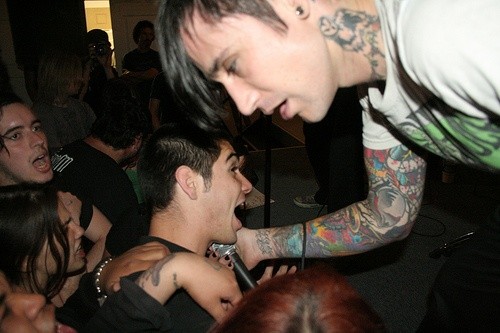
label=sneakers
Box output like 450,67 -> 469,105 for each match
293,194 -> 328,209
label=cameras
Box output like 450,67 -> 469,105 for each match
93,42 -> 107,57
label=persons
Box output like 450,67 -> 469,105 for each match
154,0 -> 500,333
0,19 -> 389,333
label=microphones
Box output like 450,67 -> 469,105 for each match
213,241 -> 259,293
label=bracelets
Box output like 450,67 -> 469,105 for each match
95,258 -> 113,298
